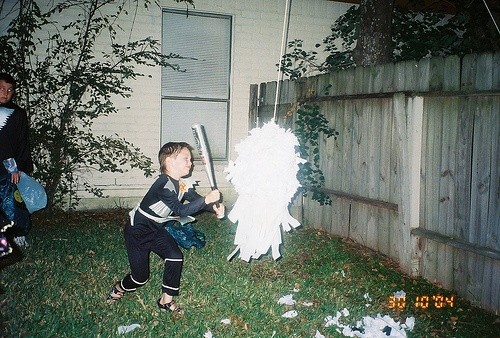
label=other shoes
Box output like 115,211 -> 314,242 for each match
13,235 -> 29,248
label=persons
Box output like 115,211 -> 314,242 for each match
105,142 -> 225,316
0,72 -> 33,249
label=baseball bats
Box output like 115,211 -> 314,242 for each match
193,123 -> 225,218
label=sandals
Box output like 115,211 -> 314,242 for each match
157,298 -> 182,316
107,281 -> 125,304
